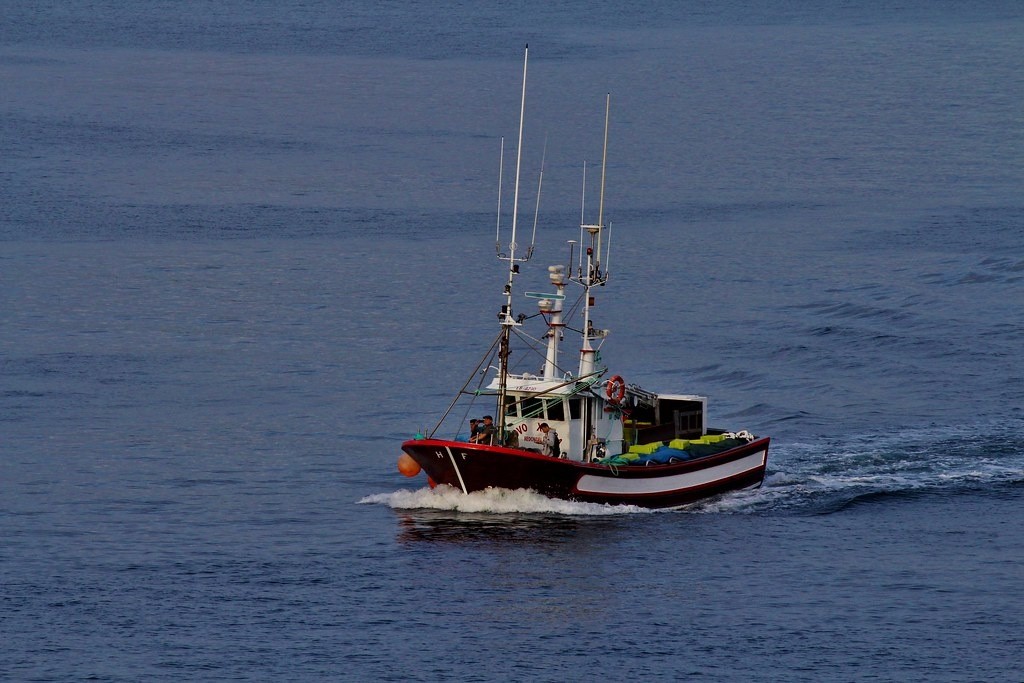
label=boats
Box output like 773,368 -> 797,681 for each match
395,41 -> 771,508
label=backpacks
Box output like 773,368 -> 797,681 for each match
550,433 -> 560,457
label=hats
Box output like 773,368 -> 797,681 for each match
539,423 -> 548,428
483,415 -> 492,421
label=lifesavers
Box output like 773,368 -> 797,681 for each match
606,375 -> 625,402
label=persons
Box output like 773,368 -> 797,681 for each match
468,415 -> 498,446
540,423 -> 560,458
